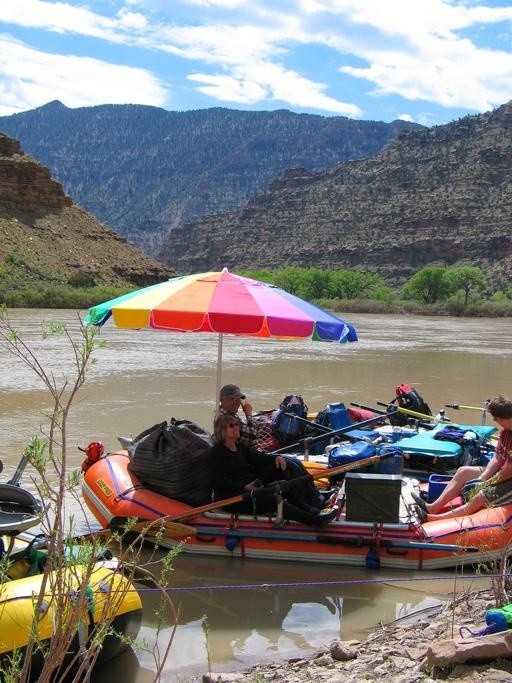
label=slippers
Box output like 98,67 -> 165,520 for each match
413,506 -> 428,523
411,492 -> 430,513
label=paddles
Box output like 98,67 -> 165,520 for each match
285,412 -> 447,476
66,452 -> 395,543
15,532 -> 157,588
119,521 -> 479,553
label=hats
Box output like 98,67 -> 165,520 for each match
219,383 -> 246,401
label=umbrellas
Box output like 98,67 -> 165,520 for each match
83,266 -> 358,422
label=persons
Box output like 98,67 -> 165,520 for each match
214,384 -> 259,449
410,396 -> 512,524
210,415 -> 338,529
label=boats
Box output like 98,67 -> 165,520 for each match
0,511 -> 145,683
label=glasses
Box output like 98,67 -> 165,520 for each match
228,422 -> 237,427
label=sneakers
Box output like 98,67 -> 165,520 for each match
320,488 -> 335,500
317,509 -> 339,524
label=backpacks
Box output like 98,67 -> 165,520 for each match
271,394 -> 308,435
